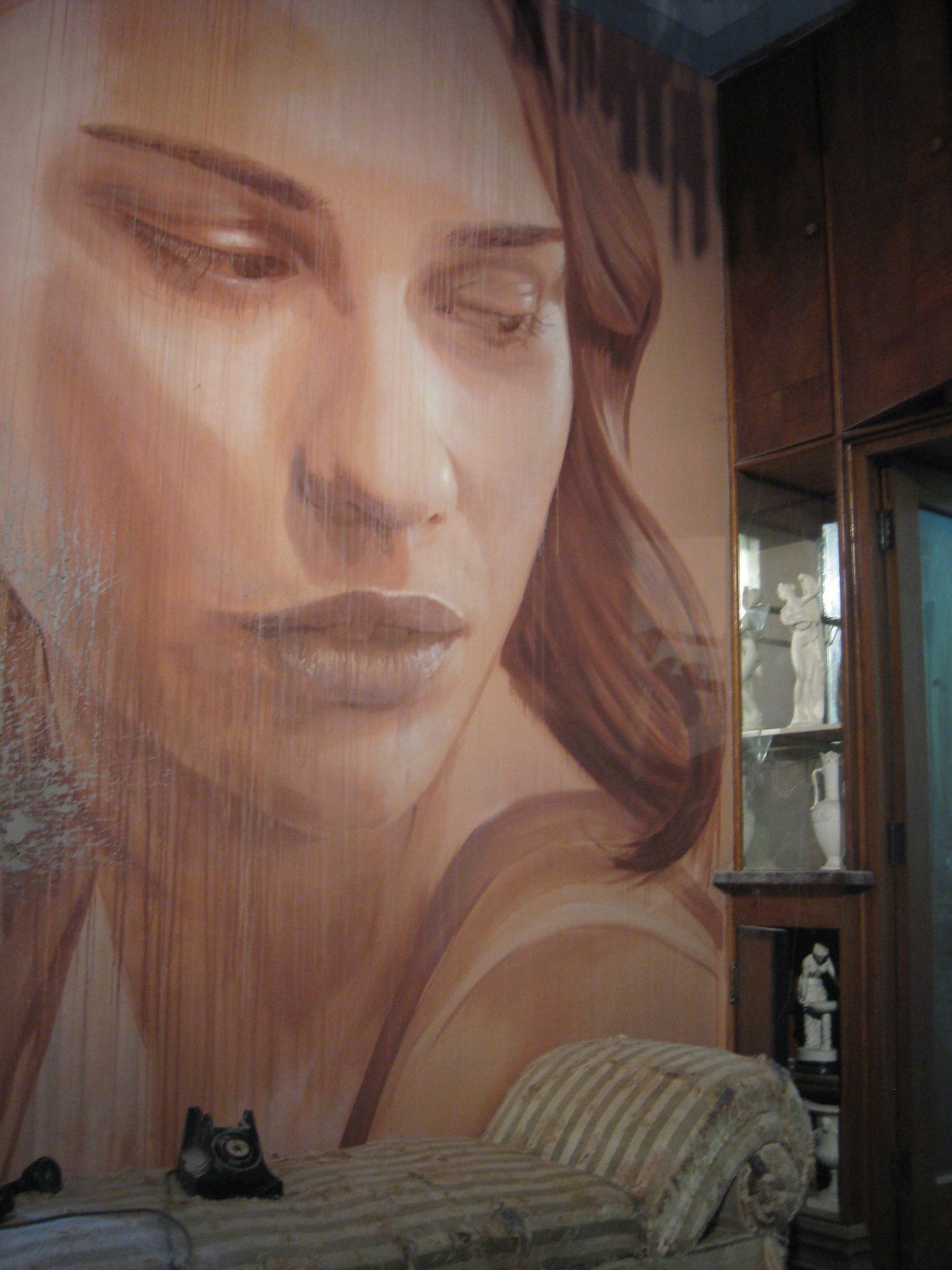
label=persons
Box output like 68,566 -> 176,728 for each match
739,586 -> 770,731
798,945 -> 837,1050
776,582 -> 824,728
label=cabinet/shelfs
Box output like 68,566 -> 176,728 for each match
774,927 -> 849,1236
736,441 -> 842,867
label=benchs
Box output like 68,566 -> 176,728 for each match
1,1036 -> 815,1270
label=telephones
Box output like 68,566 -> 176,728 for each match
0,1156 -> 64,1217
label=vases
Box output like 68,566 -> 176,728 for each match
810,748 -> 842,872
